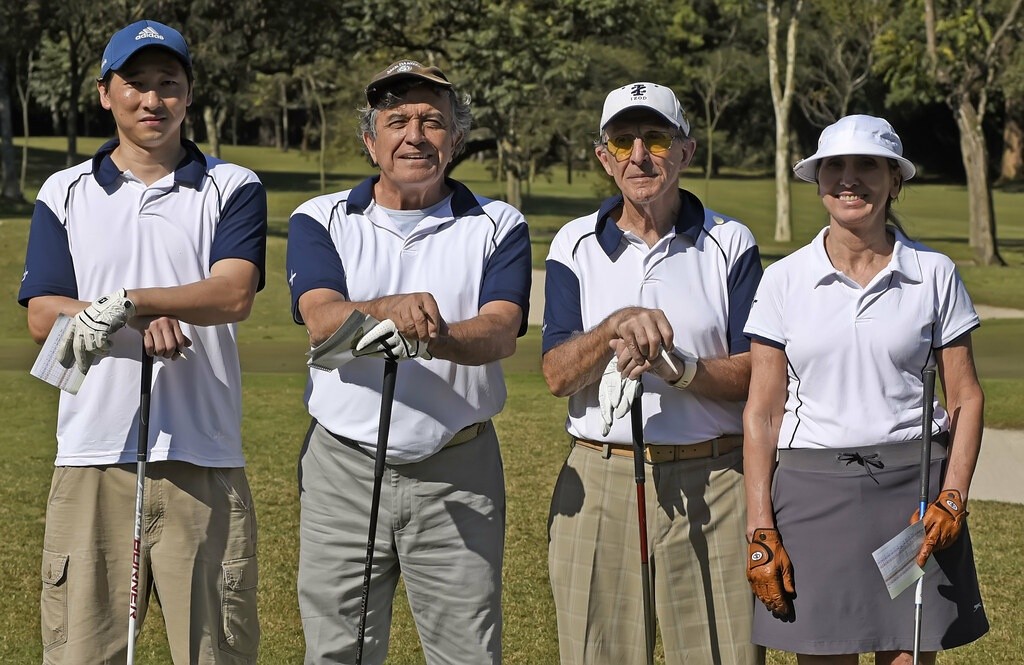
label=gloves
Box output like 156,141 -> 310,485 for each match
900,488 -> 971,566
353,317 -> 432,362
55,286 -> 138,375
741,526 -> 798,620
600,356 -> 642,438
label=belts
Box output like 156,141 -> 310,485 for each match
441,416 -> 488,450
575,438 -> 746,463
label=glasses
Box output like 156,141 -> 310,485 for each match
602,131 -> 675,156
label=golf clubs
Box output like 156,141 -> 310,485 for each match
912,367 -> 937,665
632,380 -> 658,664
122,334 -> 155,665
356,358 -> 399,664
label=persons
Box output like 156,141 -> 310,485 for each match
742,114 -> 990,665
541,81 -> 764,665
17,19 -> 267,665
285,58 -> 532,665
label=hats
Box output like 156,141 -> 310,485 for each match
365,58 -> 457,121
793,113 -> 917,184
600,81 -> 691,136
98,18 -> 196,87
